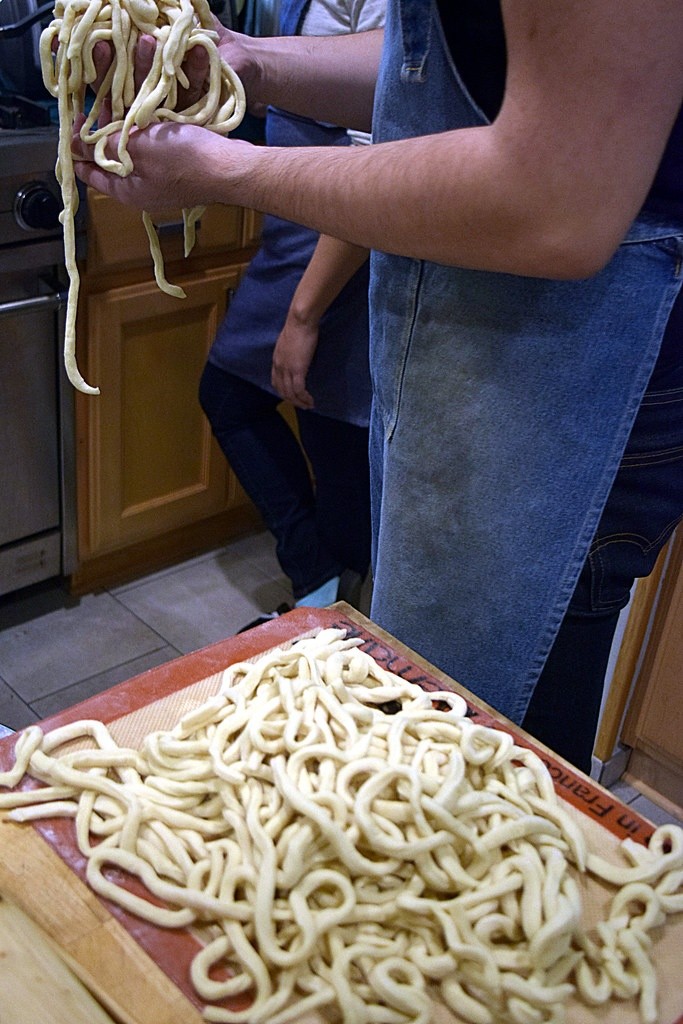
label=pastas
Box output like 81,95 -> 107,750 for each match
40,0 -> 245,395
0,626 -> 682,1024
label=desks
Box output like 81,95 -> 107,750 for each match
1,600 -> 683,1024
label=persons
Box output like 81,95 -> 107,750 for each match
197,0 -> 402,634
66,0 -> 683,787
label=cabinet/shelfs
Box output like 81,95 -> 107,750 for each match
76,186 -> 316,595
